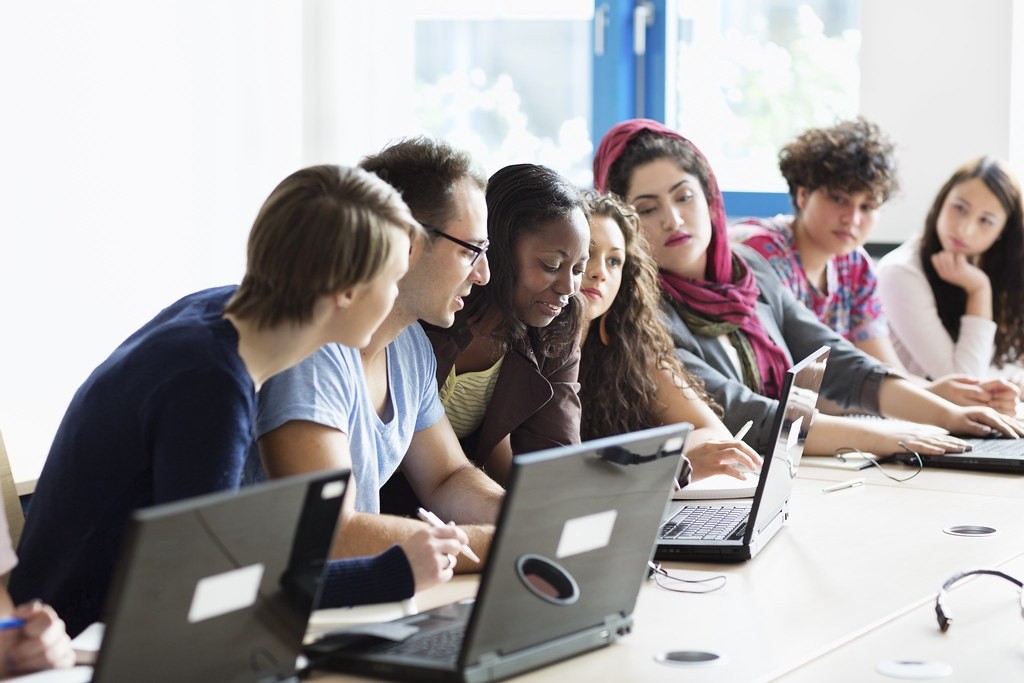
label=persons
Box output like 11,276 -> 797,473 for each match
720,117 -> 1022,421
0,117 -> 1024,674
874,154 -> 1023,382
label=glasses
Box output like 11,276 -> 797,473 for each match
418,223 -> 491,267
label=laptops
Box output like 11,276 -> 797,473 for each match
652,344 -> 830,564
90,465 -> 350,683
302,422 -> 692,683
896,432 -> 1024,471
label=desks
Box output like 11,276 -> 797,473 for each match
0,453 -> 1024,683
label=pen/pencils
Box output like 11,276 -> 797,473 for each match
414,505 -> 482,568
822,477 -> 865,493
0,617 -> 26,631
732,418 -> 755,443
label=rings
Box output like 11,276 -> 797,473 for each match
444,554 -> 452,570
